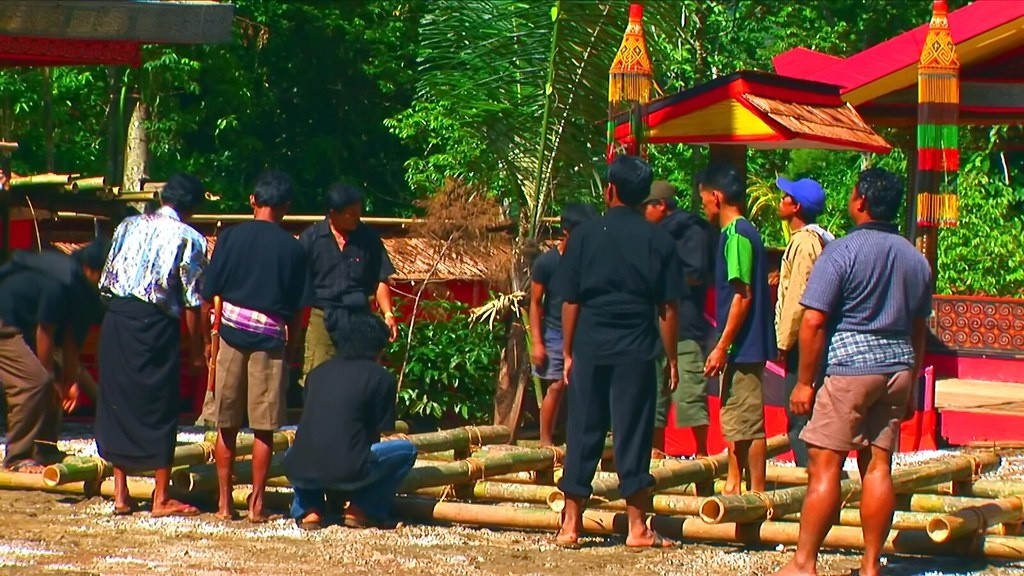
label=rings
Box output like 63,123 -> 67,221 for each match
719,371 -> 722,375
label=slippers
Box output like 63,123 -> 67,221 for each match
112,499 -> 138,514
211,510 -> 233,522
548,532 -> 581,550
297,511 -> 322,530
12,461 -> 41,474
342,511 -> 367,529
246,512 -> 268,523
625,529 -> 683,553
151,503 -> 201,518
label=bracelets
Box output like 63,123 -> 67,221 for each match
384,312 -> 394,317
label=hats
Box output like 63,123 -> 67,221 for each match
776,177 -> 826,215
643,180 -> 675,204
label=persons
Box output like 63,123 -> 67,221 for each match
96,173 -> 208,518
768,167 -> 932,576
556,156 -> 680,547
298,182 -> 398,389
0,237 -> 112,474
282,313 -> 419,530
529,167 -> 835,498
200,173 -> 307,523
